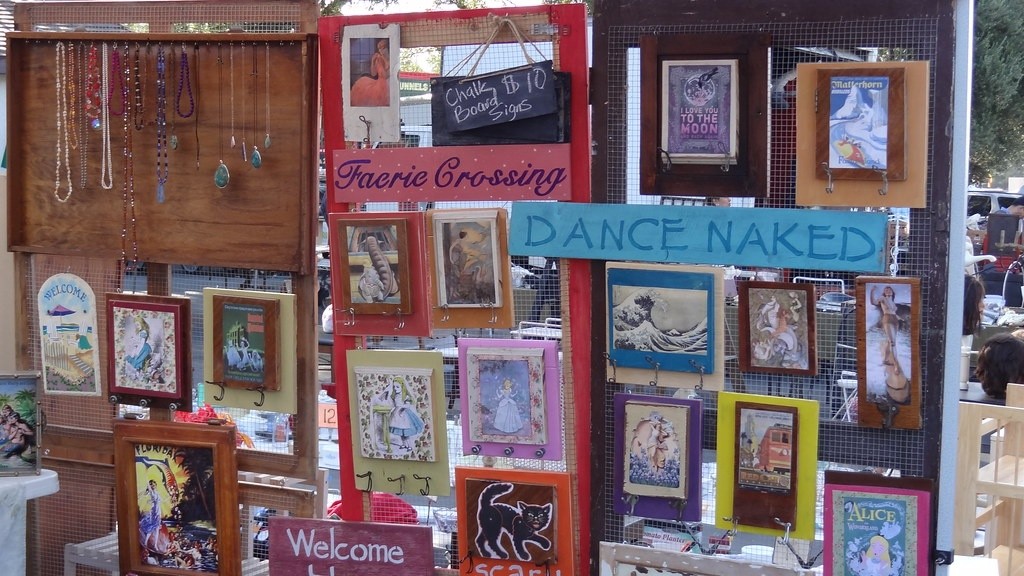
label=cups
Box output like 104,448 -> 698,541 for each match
740,545 -> 774,562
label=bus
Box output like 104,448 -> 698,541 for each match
757,424 -> 792,473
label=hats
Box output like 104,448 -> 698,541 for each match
967,214 -> 981,230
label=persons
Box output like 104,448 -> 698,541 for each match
995,198 -> 1024,220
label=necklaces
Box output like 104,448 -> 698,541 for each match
156,46 -> 169,204
54,38 -> 275,205
175,43 -> 196,118
121,46 -> 139,272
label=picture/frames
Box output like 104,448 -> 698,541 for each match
212,294 -> 281,392
336,218 -> 412,315
815,67 -> 906,182
112,417 -> 241,576
107,298 -> 183,399
429,210 -> 504,309
467,348 -> 547,444
738,280 -> 819,376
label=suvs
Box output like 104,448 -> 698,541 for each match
966,189 -> 1024,229
